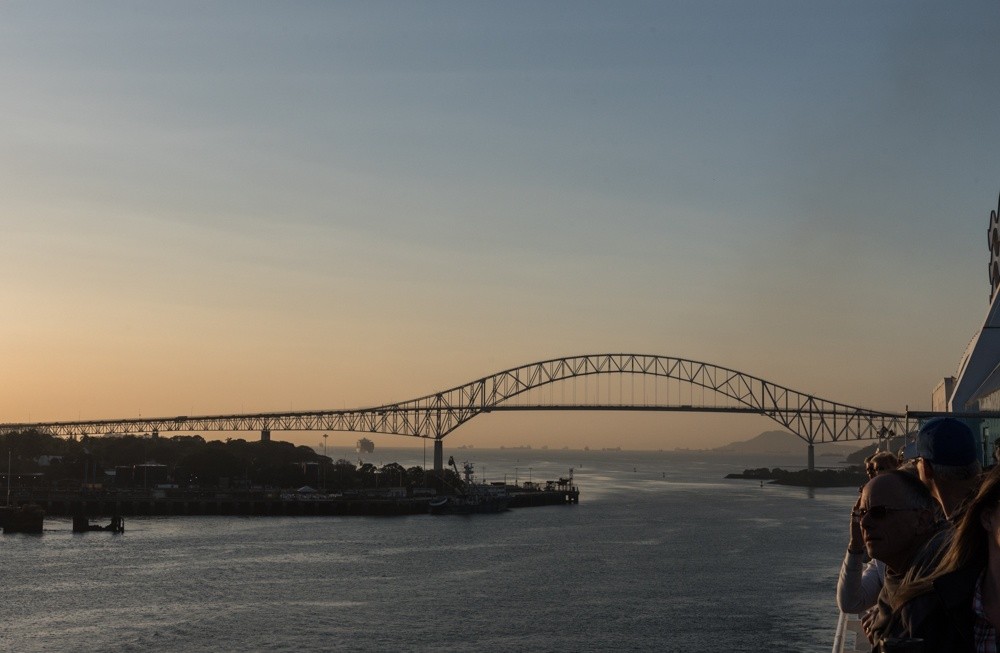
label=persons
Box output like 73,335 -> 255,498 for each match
835,418 -> 1000,614
856,468 -> 955,645
872,463 -> 1000,653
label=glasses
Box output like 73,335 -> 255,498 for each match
865,467 -> 875,472
874,466 -> 890,471
852,504 -> 922,523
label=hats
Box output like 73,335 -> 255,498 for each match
906,418 -> 978,465
